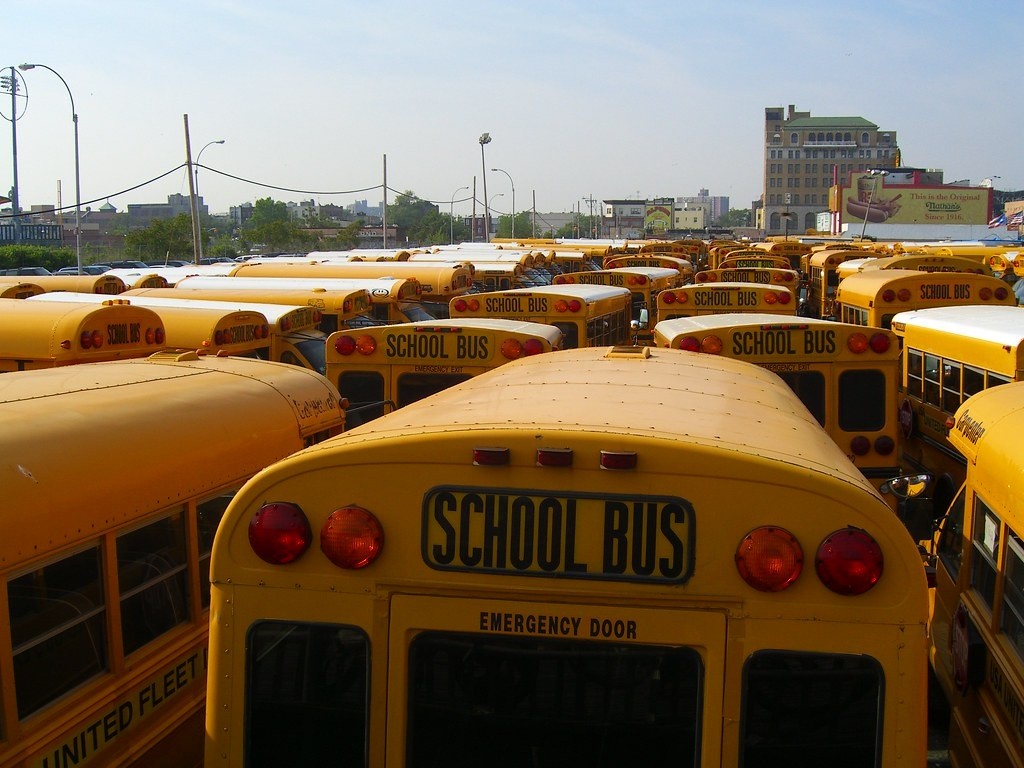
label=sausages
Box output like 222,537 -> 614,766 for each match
847,196 -> 891,222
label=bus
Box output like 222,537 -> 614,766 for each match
1,236 -> 1024,768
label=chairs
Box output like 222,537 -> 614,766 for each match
79,547 -> 187,656
7,584 -> 104,720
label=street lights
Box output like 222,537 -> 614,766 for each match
18,62 -> 84,274
195,139 -> 226,260
479,133 -> 493,242
489,168 -> 515,238
487,193 -> 503,241
449,185 -> 469,244
